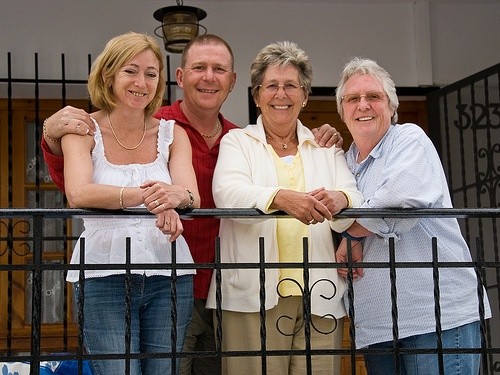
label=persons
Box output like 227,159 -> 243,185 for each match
335,57 -> 492,375
61,31 -> 202,375
205,42 -> 362,375
41,35 -> 343,375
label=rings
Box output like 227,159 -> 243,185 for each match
307,219 -> 315,225
166,218 -> 170,225
155,200 -> 159,206
334,133 -> 338,139
77,120 -> 81,128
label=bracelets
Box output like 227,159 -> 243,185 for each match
120,188 -> 124,209
342,231 -> 365,241
43,118 -> 61,143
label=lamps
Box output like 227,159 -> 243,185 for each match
153,0 -> 208,54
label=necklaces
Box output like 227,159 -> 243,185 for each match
107,110 -> 146,149
265,134 -> 295,150
200,118 -> 221,138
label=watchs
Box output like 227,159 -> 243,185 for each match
177,190 -> 194,210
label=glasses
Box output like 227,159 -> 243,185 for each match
340,92 -> 388,106
259,80 -> 304,91
